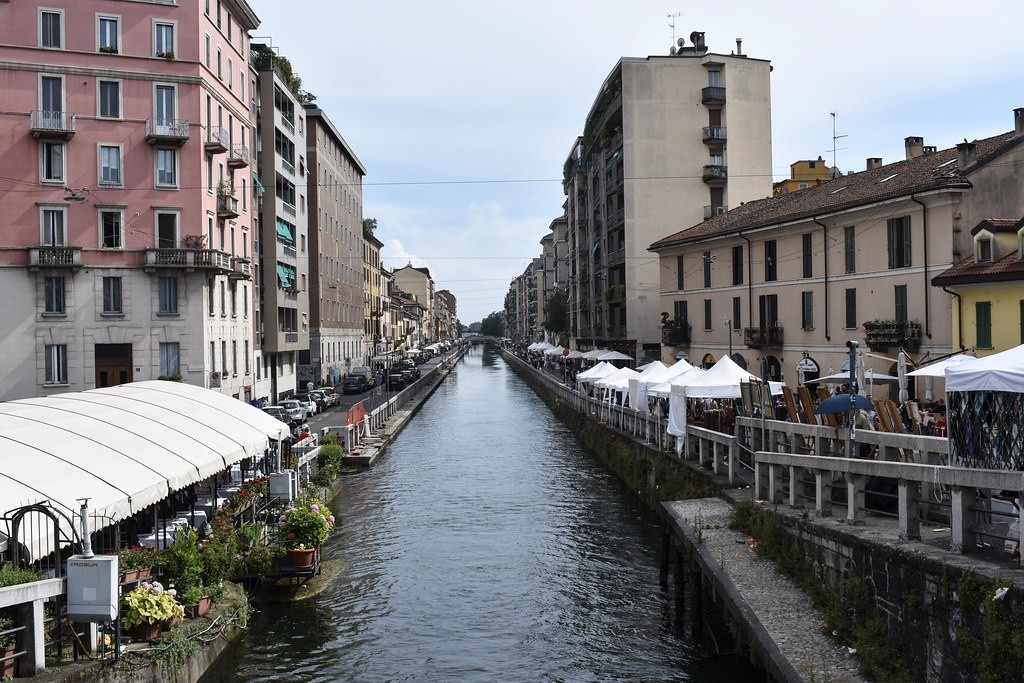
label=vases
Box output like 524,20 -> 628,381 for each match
132,622 -> 162,640
137,568 -> 151,577
166,617 -> 182,630
286,545 -> 315,567
122,571 -> 138,582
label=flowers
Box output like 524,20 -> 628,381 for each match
121,579 -> 185,625
106,543 -> 162,569
209,475 -> 335,549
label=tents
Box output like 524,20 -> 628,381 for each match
527,342 -> 634,369
576,354 -> 785,421
0,380 -> 291,566
944,343 -> 1024,466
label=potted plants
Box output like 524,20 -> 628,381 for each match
168,525 -> 213,616
319,445 -> 343,479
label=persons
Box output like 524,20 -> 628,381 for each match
835,386 -> 842,395
250,397 -> 268,409
307,381 -> 313,392
854,408 -> 879,458
933,399 -> 947,437
841,384 -> 848,394
297,427 -> 314,442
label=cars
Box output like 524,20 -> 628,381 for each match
343,364 -> 421,395
261,406 -> 292,424
311,393 -> 327,414
310,390 -> 332,408
278,400 -> 308,425
291,393 -> 317,417
319,386 -> 341,406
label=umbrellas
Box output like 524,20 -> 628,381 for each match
814,394 -> 874,424
904,354 -> 978,378
802,349 -> 912,404
